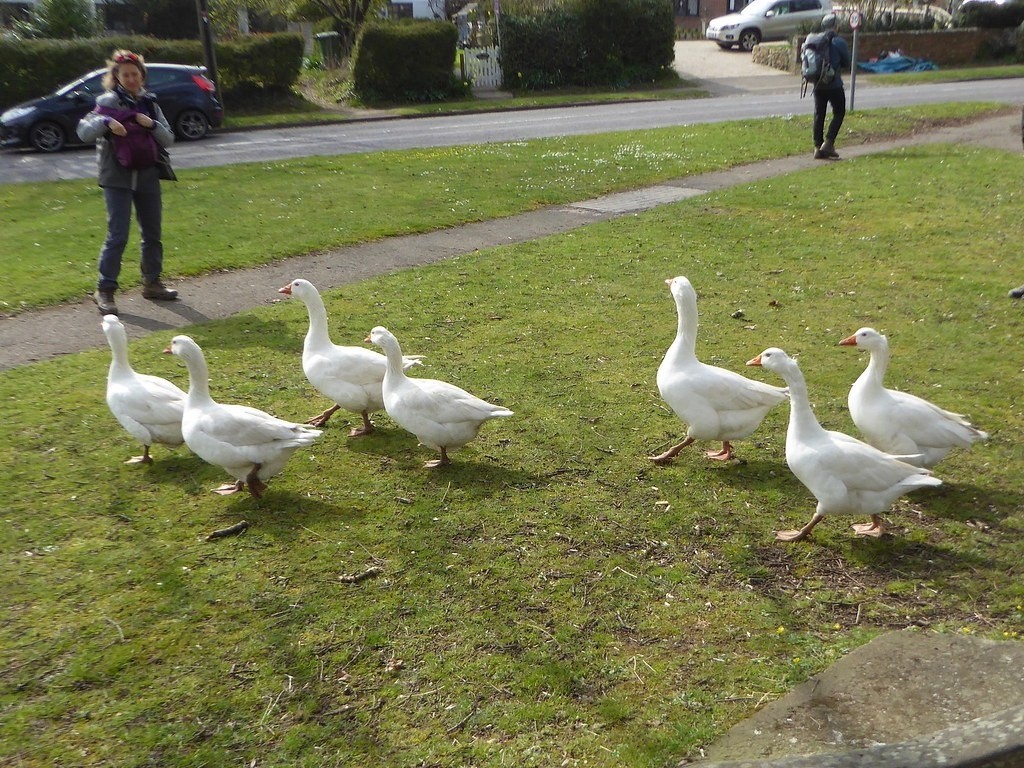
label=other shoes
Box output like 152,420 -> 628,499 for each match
819,139 -> 839,158
813,146 -> 826,158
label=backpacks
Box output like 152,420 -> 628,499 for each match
799,31 -> 835,85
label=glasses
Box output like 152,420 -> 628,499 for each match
113,52 -> 138,63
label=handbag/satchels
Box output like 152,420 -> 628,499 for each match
92,105 -> 157,169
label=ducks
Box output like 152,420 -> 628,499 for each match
642,276 -> 792,463
162,334 -> 324,499
747,346 -> 943,541
280,276 -> 428,437
839,326 -> 989,472
364,327 -> 514,468
101,315 -> 189,464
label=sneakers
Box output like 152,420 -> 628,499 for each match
141,278 -> 178,300
92,288 -> 119,315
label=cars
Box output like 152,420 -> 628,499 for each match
1,63 -> 224,154
706,0 -> 834,52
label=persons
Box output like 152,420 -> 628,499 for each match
76,49 -> 179,314
805,23 -> 818,41
809,13 -> 850,159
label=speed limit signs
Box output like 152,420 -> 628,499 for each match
849,11 -> 861,28
